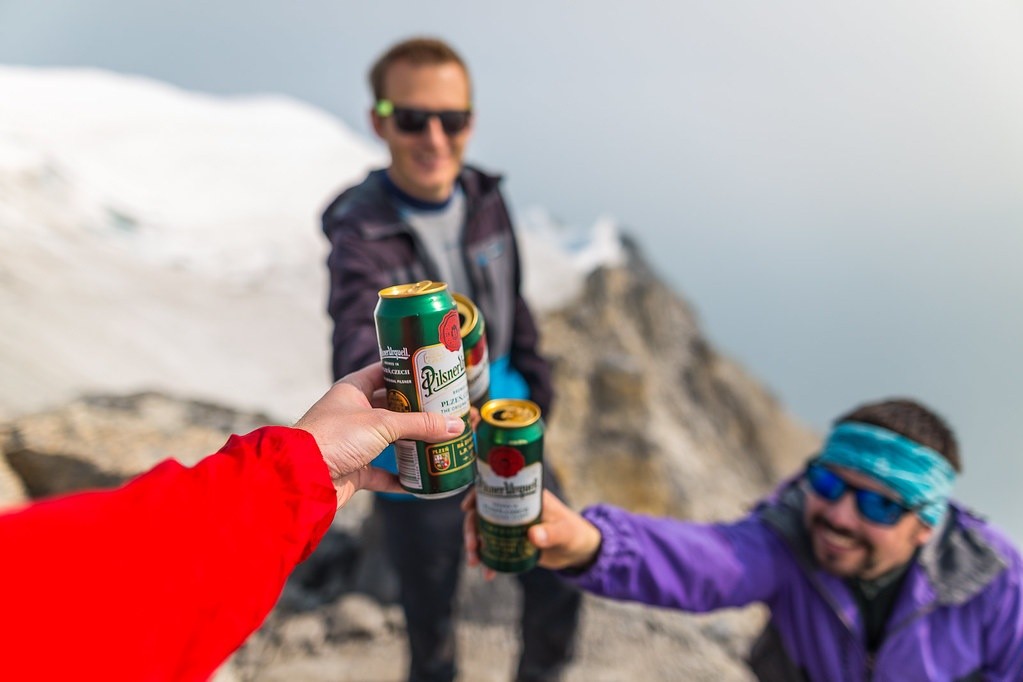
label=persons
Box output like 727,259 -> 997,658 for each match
319,35 -> 592,682
461,395 -> 1022,682
1,359 -> 481,681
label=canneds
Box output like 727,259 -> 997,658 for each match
450,291 -> 491,406
375,281 -> 480,500
475,398 -> 546,574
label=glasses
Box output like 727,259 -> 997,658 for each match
390,105 -> 472,135
805,457 -> 928,528
